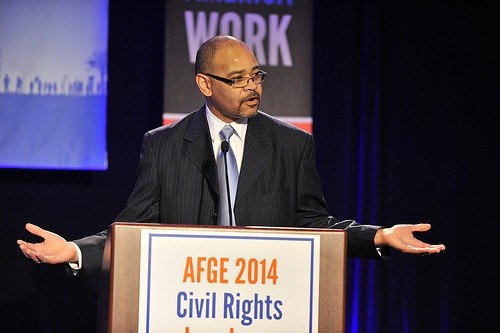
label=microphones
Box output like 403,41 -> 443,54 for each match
221,141 -> 232,226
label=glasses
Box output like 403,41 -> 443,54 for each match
205,70 -> 267,87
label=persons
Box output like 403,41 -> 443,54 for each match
17,34 -> 445,277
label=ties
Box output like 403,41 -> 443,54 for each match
217,126 -> 239,226
